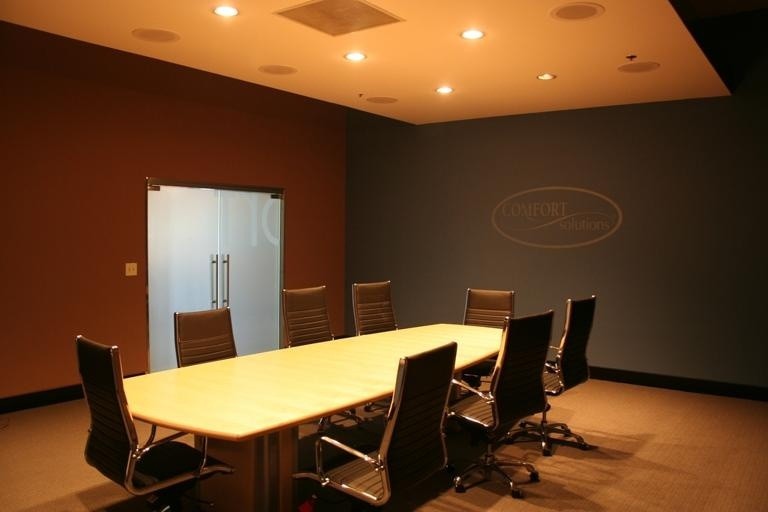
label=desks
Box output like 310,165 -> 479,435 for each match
123,324 -> 503,512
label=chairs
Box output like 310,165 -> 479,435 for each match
506,295 -> 597,457
282,285 -> 334,348
442,309 -> 555,498
174,307 -> 238,368
462,288 -> 515,330
352,280 -> 399,336
298,342 -> 458,506
75,335 -> 236,511
